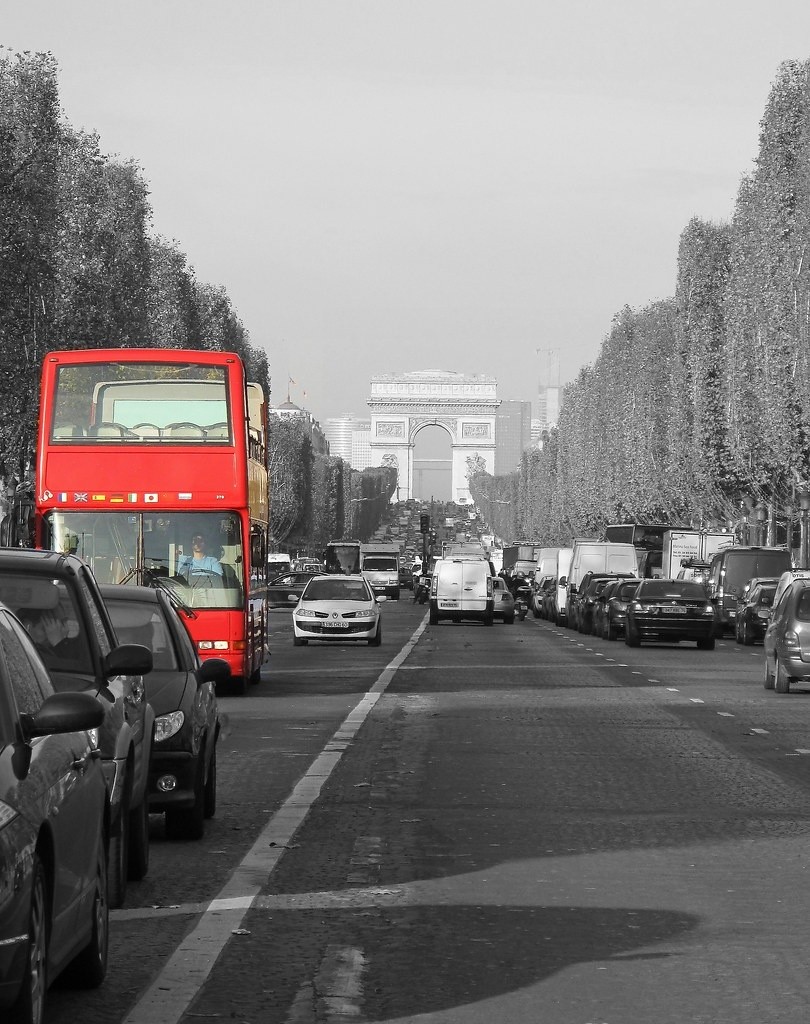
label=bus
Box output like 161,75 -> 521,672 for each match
325,540 -> 363,579
266,553 -> 290,584
35,348 -> 271,697
290,557 -> 320,572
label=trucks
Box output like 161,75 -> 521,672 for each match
503,523 -> 735,626
360,543 -> 400,600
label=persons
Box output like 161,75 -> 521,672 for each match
413,568 -> 431,604
498,568 -> 530,602
177,533 -> 223,577
12,607 -> 66,660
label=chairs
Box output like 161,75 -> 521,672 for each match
52,421 -> 230,442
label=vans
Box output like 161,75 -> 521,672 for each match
706,546 -> 797,635
442,542 -> 486,559
429,552 -> 494,626
303,563 -> 326,573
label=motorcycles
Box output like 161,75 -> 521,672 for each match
414,577 -> 432,605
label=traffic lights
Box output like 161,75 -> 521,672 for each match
420,515 -> 431,533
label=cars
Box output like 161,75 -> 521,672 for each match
293,575 -> 381,647
491,577 -> 516,625
0,547 -> 157,908
569,571 -> 637,630
369,498 -> 506,590
267,570 -> 330,611
623,579 -> 716,648
735,571 -> 810,693
593,581 -> 619,637
97,583 -> 231,841
578,578 -> 615,634
0,601 -> 111,1023
608,578 -> 652,641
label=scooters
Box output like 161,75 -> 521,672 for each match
512,586 -> 531,623
